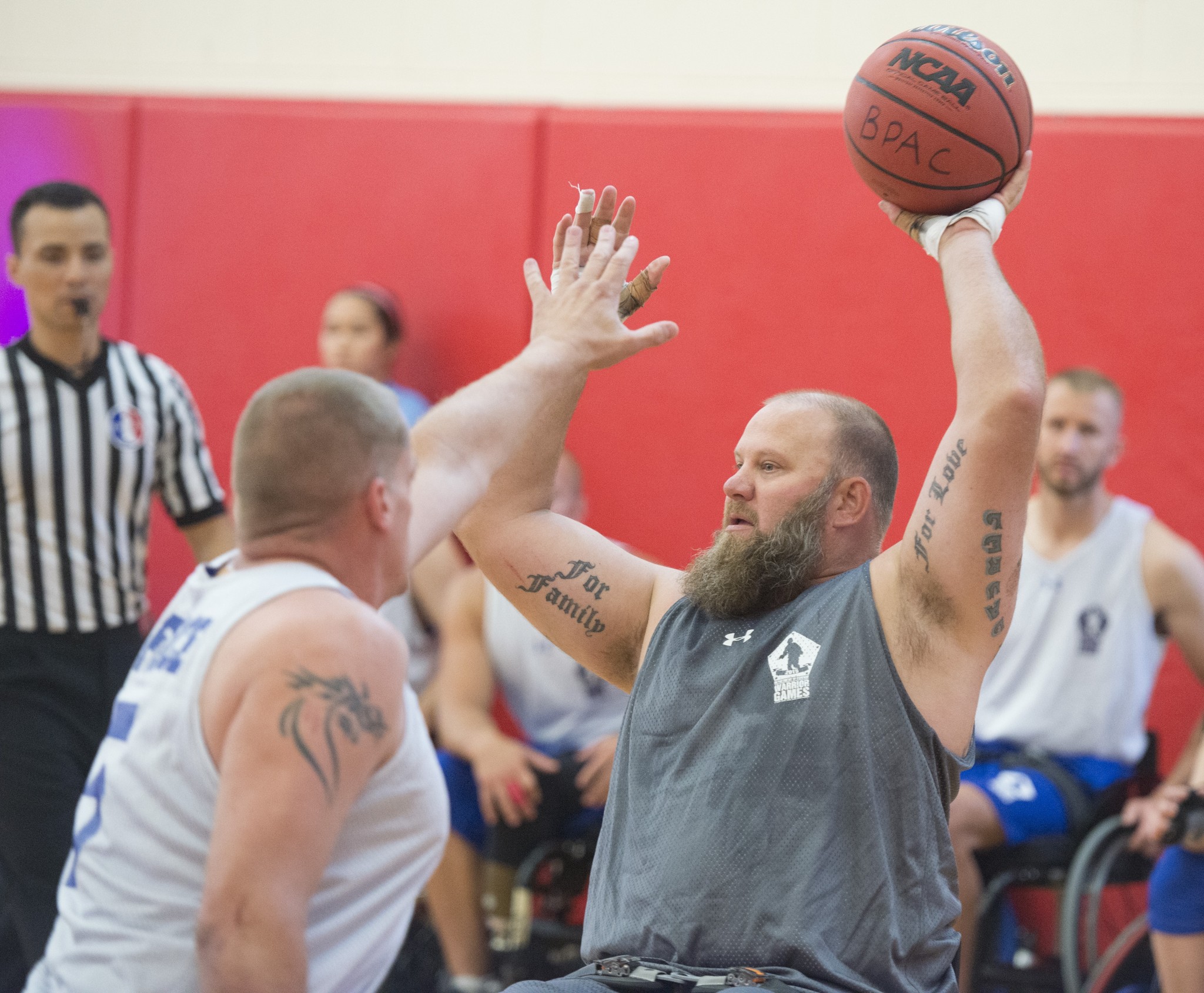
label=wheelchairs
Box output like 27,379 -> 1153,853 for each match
479,759 -> 599,989
970,790 -> 1204,993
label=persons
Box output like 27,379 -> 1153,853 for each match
951,370 -> 1204,993
0,182 -> 232,993
453,148 -> 1045,993
29,222 -> 677,993
315,280 -> 666,993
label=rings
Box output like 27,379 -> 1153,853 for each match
628,268 -> 658,308
615,231 -> 629,249
589,217 -> 612,244
575,189 -> 595,214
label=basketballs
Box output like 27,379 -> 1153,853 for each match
843,24 -> 1035,212
